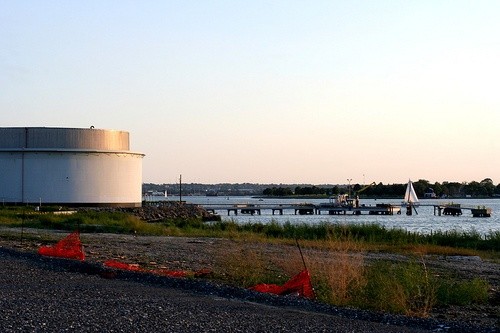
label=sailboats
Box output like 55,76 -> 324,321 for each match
401,178 -> 420,208
